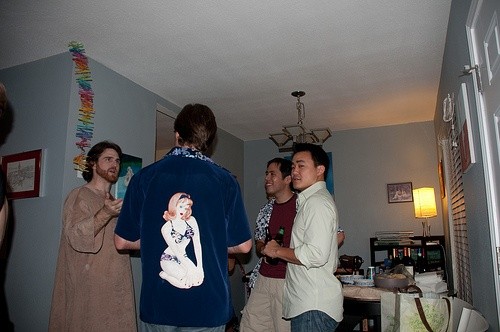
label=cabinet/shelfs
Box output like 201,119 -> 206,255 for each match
371,235 -> 444,281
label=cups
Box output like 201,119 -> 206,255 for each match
367,267 -> 376,280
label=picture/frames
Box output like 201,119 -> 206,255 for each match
2,150 -> 41,199
387,182 -> 413,203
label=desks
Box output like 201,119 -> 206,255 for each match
344,284 -> 389,332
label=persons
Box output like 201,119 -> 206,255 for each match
264,143 -> 344,332
47,141 -> 139,332
390,187 -> 411,201
238,157 -> 345,332
114,103 -> 252,332
0,83 -> 16,332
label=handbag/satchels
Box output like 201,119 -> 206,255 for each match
338,254 -> 364,273
381,284 -> 454,332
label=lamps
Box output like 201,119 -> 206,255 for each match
268,90 -> 332,152
412,187 -> 437,237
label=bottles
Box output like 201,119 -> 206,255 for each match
390,248 -> 428,279
266,226 -> 285,266
264,226 -> 273,264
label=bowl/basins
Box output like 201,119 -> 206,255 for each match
353,279 -> 374,286
340,275 -> 364,284
